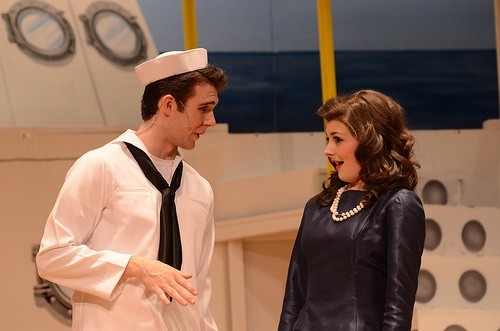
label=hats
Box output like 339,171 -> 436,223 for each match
135,48 -> 208,86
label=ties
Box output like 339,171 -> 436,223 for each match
123,142 -> 184,302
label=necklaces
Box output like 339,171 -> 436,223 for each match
329,182 -> 379,223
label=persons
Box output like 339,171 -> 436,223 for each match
277,88 -> 427,331
35,47 -> 229,331
